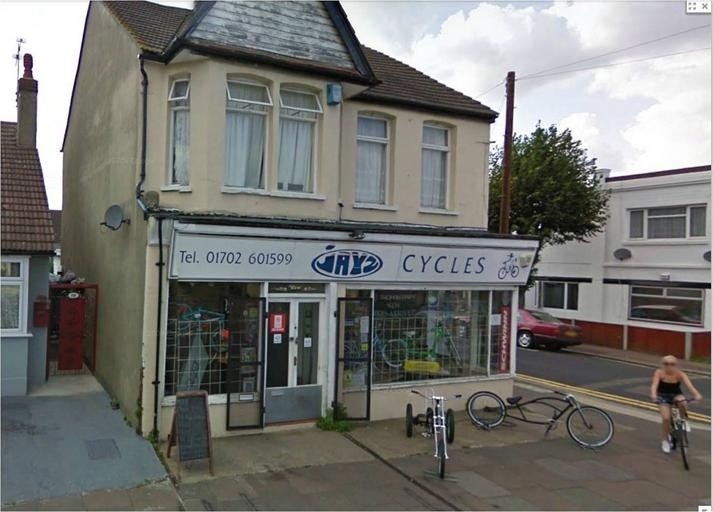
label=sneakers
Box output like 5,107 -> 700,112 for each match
682,422 -> 691,432
661,441 -> 670,453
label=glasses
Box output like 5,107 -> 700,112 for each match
663,362 -> 676,367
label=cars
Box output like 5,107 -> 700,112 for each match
517,309 -> 585,352
631,304 -> 701,325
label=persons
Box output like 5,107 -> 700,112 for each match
650,355 -> 703,455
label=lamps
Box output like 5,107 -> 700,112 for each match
348,229 -> 366,240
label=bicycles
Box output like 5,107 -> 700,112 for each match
343,318 -> 464,373
168,298 -> 235,353
466,390 -> 614,450
654,397 -> 696,471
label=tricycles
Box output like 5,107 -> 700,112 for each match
405,390 -> 462,479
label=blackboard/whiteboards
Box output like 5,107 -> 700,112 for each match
176,391 -> 212,462
168,409 -> 177,447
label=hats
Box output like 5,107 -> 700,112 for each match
662,355 -> 677,361
